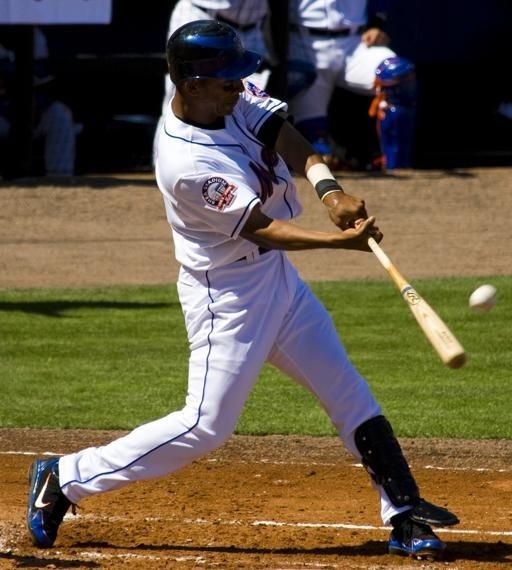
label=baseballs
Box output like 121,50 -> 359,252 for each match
469,285 -> 498,314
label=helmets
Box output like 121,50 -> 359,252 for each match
165,18 -> 264,81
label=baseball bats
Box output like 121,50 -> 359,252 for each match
354,217 -> 465,368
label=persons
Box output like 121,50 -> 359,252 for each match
0,23 -> 80,178
160,1 -> 282,118
279,0 -> 417,183
23,18 -> 454,561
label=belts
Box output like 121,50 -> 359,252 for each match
235,246 -> 272,262
291,22 -> 368,38
215,13 -> 256,33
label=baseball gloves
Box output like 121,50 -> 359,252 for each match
312,135 -> 346,170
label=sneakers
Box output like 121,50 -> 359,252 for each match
27,457 -> 71,548
388,517 -> 451,562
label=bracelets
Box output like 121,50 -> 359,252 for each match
312,178 -> 343,200
305,162 -> 344,202
